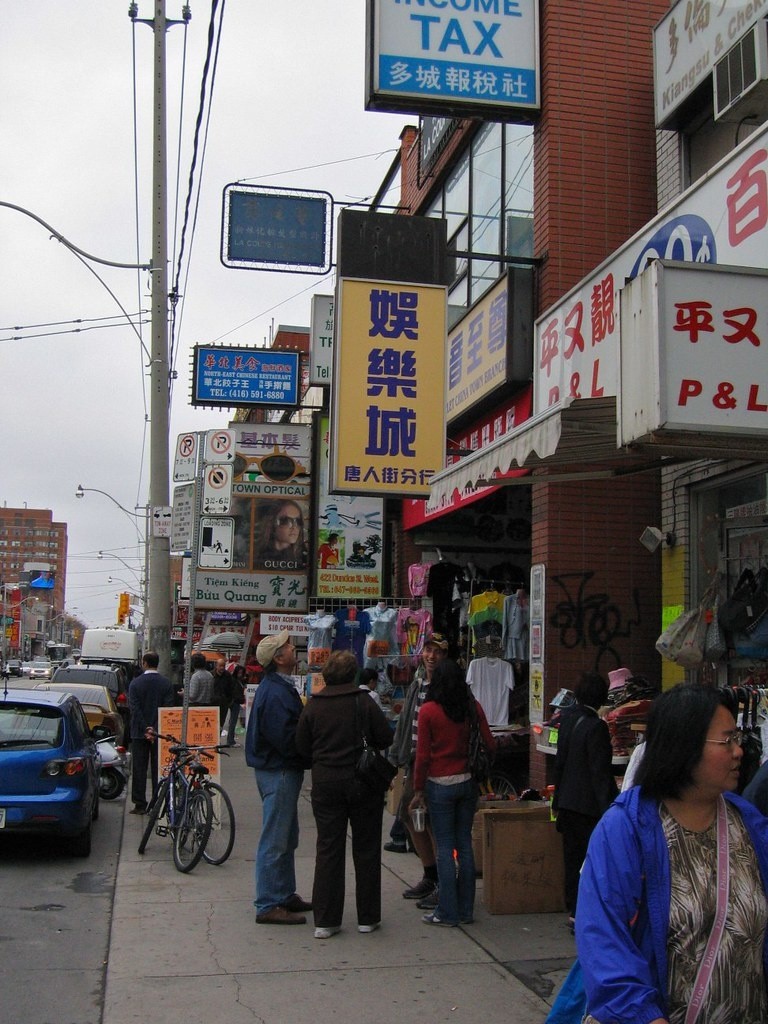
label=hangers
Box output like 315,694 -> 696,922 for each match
484,578 -> 498,597
516,580 -> 529,599
501,579 -> 515,596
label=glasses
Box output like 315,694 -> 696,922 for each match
705,731 -> 742,750
276,515 -> 304,530
233,452 -> 306,483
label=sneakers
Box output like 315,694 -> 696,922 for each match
279,893 -> 312,912
256,906 -> 307,924
461,916 -> 473,924
421,913 -> 455,927
403,878 -> 438,899
415,883 -> 439,908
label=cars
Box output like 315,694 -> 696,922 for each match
5,649 -> 81,679
0,684 -> 129,857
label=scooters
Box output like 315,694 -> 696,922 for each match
92,726 -> 133,800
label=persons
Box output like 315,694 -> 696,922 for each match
333,606 -> 374,668
128,651 -> 180,815
304,609 -> 338,648
389,632 -> 448,909
245,628 -> 312,924
551,675 -> 619,923
177,652 -> 244,747
3,661 -> 10,680
256,499 -> 307,571
0,657 -> 4,680
413,658 -> 499,928
295,651 -> 393,938
358,669 -> 387,806
319,502 -> 380,568
545,682 -> 768,1024
362,602 -> 402,695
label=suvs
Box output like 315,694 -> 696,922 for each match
51,662 -> 132,725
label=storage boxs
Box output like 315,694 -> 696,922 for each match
533,724 -> 557,749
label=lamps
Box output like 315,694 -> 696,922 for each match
639,527 -> 677,553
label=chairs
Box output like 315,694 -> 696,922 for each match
28,713 -> 56,730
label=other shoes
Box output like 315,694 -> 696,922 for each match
315,925 -> 341,938
129,808 -> 145,815
384,841 -> 407,853
409,847 -> 415,852
231,743 -> 240,748
569,912 -> 575,921
358,922 -> 380,933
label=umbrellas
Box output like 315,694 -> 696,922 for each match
733,686 -> 739,796
193,631 -> 246,664
747,687 -> 763,779
738,687 -> 751,791
191,650 -> 227,662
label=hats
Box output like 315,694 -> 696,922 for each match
607,667 -> 633,690
256,629 -> 289,669
423,633 -> 448,653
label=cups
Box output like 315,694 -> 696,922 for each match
411,808 -> 425,832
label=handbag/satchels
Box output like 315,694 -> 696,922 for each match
655,565 -> 767,667
356,749 -> 398,792
466,694 -> 500,781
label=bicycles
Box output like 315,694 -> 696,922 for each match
138,725 -> 235,873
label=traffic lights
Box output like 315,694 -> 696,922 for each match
118,607 -> 126,625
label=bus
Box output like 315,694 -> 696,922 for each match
49,644 -> 71,661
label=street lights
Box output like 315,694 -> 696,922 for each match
22,605 -> 53,659
75,484 -> 150,656
3,583 -> 39,658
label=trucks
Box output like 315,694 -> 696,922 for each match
79,625 -> 139,683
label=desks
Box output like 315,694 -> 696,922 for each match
536,743 -> 631,765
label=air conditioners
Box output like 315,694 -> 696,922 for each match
713,18 -> 768,126
